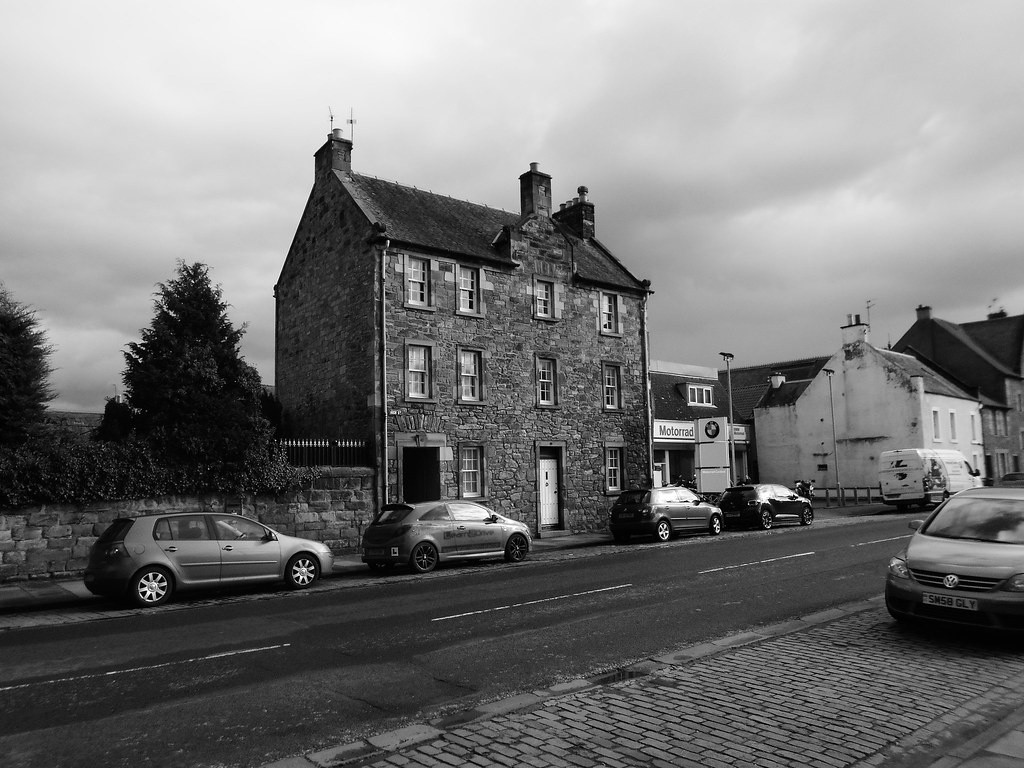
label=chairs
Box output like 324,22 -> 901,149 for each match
160,527 -> 202,540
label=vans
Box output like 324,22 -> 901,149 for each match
877,447 -> 985,512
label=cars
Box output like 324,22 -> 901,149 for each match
717,483 -> 815,531
361,498 -> 533,574
999,472 -> 1024,489
83,510 -> 335,609
609,486 -> 726,544
885,486 -> 1024,632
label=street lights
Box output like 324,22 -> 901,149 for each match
719,351 -> 738,487
824,368 -> 842,504
